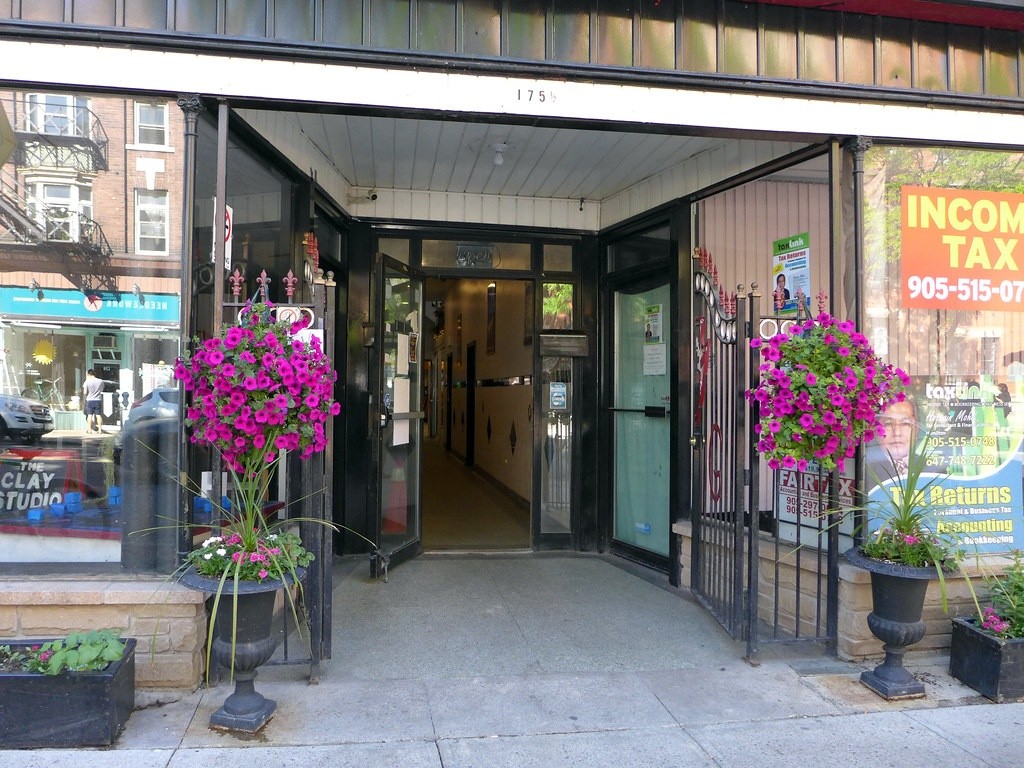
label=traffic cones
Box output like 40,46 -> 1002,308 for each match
381,455 -> 407,544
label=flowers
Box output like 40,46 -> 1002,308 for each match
774,421 -> 1020,623
741,313 -> 911,472
172,299 -> 341,480
972,547 -> 1024,640
128,426 -> 380,691
0,627 -> 126,675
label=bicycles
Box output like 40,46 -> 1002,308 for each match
21,377 -> 66,412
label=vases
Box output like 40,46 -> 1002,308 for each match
176,567 -> 307,672
947,615 -> 1024,704
0,638 -> 137,752
843,546 -> 957,648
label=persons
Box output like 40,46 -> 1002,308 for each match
82,368 -> 105,435
866,395 -> 947,492
645,324 -> 652,337
773,274 -> 790,302
993,383 -> 1012,418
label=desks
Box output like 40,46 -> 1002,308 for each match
0,500 -> 284,563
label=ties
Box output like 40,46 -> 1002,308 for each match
893,453 -> 908,475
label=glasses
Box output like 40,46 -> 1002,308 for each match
882,420 -> 913,431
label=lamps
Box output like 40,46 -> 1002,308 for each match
131,285 -> 142,297
27,277 -> 43,292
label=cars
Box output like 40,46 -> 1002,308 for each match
128,387 -> 180,424
0,394 -> 54,445
